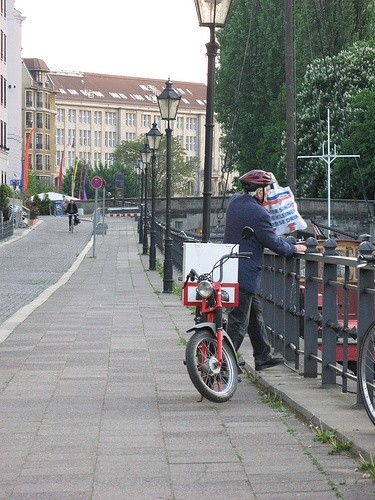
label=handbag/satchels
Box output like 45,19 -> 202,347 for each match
74,215 -> 79,225
263,171 -> 308,236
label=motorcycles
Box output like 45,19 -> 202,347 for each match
180,226 -> 255,404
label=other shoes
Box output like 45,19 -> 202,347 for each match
255,356 -> 285,371
212,375 -> 242,384
69,228 -> 71,231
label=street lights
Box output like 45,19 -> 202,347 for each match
146,121 -> 163,271
195,0 -> 233,244
136,143 -> 153,256
156,75 -> 182,293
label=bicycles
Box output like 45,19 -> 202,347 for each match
358,320 -> 375,427
66,212 -> 78,233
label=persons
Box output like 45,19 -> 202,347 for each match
62,196 -> 70,205
221,170 -> 307,372
65,199 -> 79,231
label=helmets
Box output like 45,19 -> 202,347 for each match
238,169 -> 276,191
